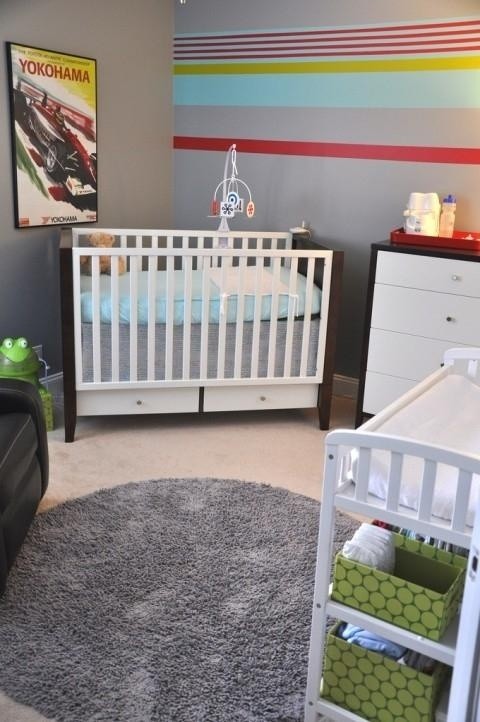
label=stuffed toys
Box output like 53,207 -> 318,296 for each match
80,232 -> 125,276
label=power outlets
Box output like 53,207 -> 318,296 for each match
32,345 -> 43,365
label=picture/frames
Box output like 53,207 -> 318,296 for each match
5,41 -> 98,228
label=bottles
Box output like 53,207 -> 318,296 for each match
438,194 -> 458,239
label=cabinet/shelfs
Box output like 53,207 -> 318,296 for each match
304,347 -> 480,722
355,239 -> 480,432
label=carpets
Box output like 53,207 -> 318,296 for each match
1,476 -> 364,722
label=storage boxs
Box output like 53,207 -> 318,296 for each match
370,519 -> 470,603
330,545 -> 466,641
321,620 -> 446,722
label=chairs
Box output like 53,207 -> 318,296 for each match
0,378 -> 49,600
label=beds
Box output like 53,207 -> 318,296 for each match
58,215 -> 345,443
